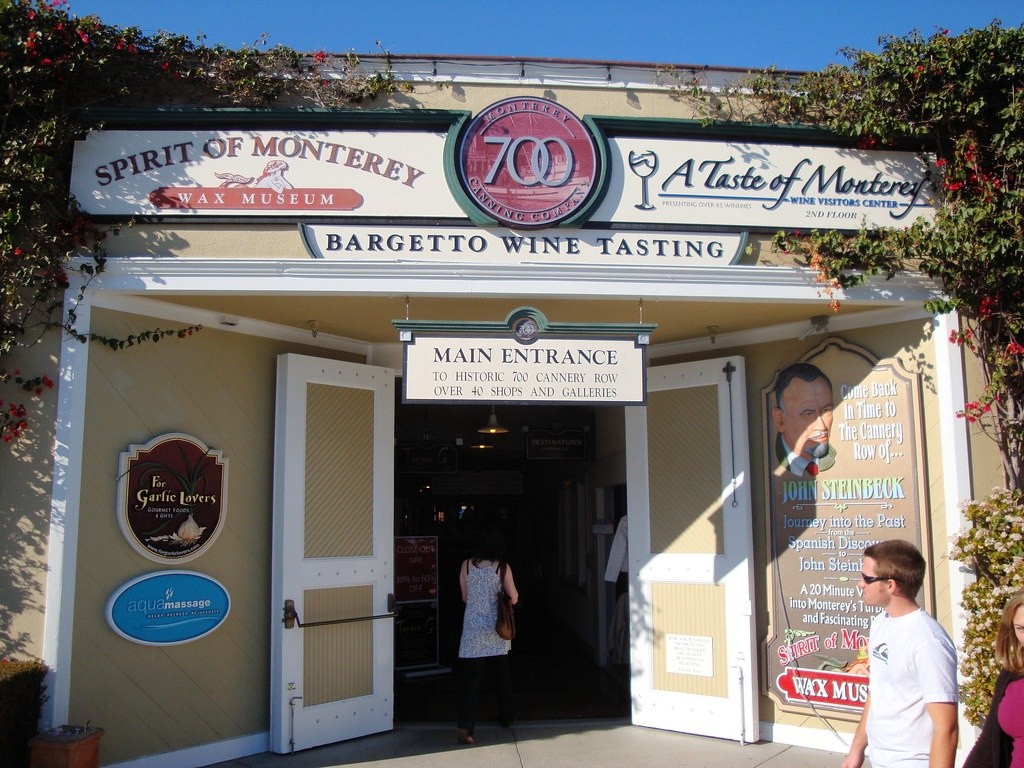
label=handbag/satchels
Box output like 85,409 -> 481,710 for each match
496,559 -> 515,640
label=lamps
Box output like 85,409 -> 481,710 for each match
468,434 -> 496,448
477,405 -> 509,434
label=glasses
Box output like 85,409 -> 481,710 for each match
861,569 -> 909,585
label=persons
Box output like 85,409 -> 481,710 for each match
963,586 -> 1024,768
841,539 -> 958,767
456,526 -> 518,744
